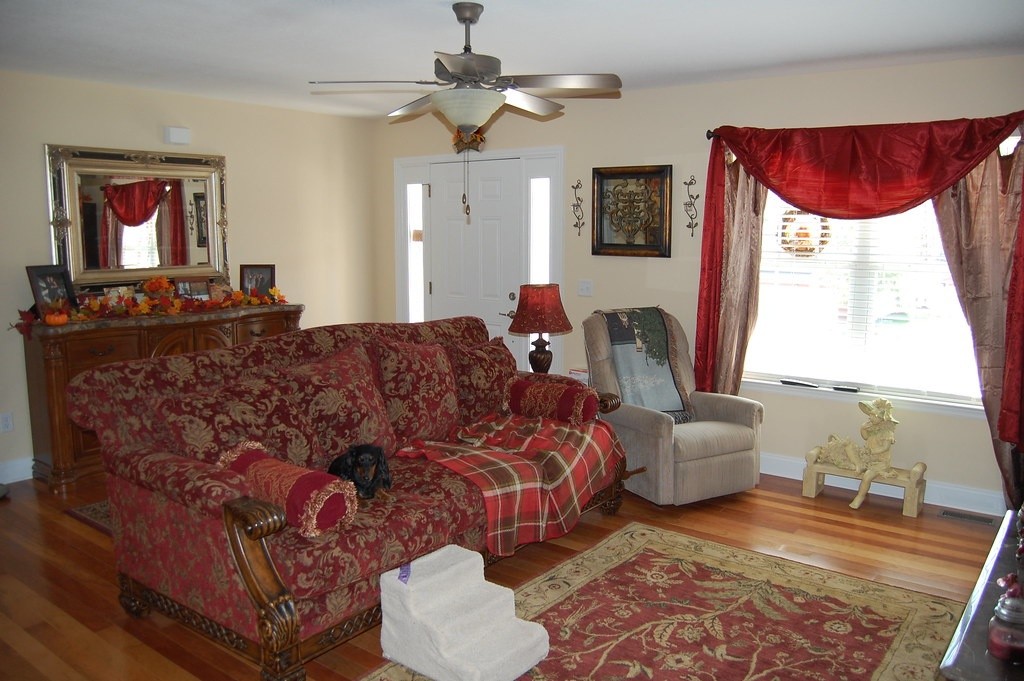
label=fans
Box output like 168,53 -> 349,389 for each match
308,2 -> 623,118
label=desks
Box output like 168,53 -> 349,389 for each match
940,508 -> 1024,681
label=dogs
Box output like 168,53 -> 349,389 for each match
328,444 -> 393,500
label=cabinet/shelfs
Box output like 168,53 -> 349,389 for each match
21,303 -> 306,499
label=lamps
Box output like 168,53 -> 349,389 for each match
507,283 -> 573,373
430,88 -> 507,215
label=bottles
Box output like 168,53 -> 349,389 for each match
1016,500 -> 1024,589
987,586 -> 1023,664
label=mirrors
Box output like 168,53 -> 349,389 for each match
43,144 -> 231,294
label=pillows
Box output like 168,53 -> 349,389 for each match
503,378 -> 601,425
277,341 -> 399,459
217,442 -> 358,539
368,336 -> 463,448
445,337 -> 517,425
146,366 -> 326,470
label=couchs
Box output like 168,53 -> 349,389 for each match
581,305 -> 765,507
65,317 -> 628,681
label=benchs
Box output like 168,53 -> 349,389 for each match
801,447 -> 927,518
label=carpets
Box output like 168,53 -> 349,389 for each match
357,521 -> 965,681
65,501 -> 111,540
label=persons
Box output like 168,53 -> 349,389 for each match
46,276 -> 60,303
844,398 -> 900,509
250,273 -> 264,290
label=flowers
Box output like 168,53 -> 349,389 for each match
9,276 -> 288,340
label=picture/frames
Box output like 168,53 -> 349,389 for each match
192,193 -> 207,248
592,164 -> 673,257
240,264 -> 276,301
174,276 -> 213,303
26,264 -> 80,319
